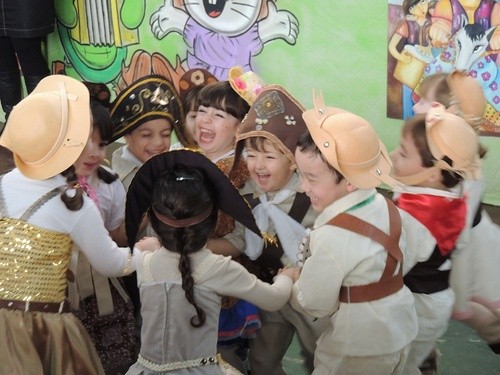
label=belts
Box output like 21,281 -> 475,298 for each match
0,299 -> 72,314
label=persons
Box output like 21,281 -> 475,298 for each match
124,149 -> 301,375
0,75 -> 141,375
76,64 -> 500,374
388,104 -> 484,375
0,0 -> 55,135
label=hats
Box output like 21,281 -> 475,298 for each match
446,69 -> 486,130
228,66 -> 265,107
425,100 -> 483,179
302,88 -> 393,191
0,74 -> 92,182
